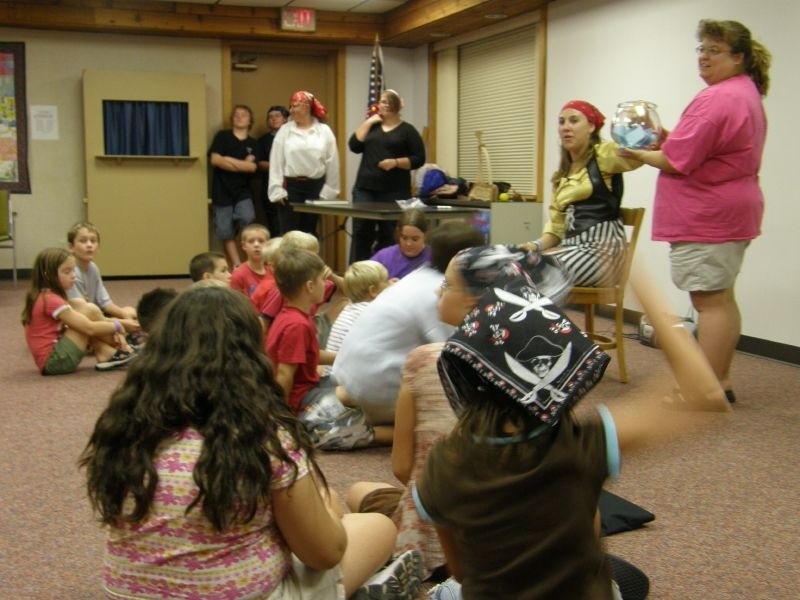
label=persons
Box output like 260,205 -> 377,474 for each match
413,262 -> 731,600
207,105 -> 259,272
66,222 -> 147,348
21,247 -> 142,375
77,278 -> 427,600
257,105 -> 289,225
616,20 -> 770,405
389,249 -> 503,578
267,91 -> 341,234
348,89 -> 426,265
137,210 -> 487,449
509,100 -> 665,308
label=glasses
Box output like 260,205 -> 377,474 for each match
696,45 -> 734,56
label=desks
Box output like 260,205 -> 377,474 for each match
289,201 -> 492,249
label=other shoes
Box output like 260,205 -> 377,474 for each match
663,385 -> 735,411
350,550 -> 424,600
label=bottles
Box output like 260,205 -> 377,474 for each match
610,99 -> 663,150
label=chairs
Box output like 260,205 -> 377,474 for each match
565,207 -> 645,383
1,188 -> 19,288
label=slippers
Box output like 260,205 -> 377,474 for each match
96,349 -> 138,369
125,333 -> 145,349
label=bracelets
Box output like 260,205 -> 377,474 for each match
530,239 -> 544,252
395,159 -> 399,168
113,321 -> 121,333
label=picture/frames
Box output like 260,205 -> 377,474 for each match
0,40 -> 33,195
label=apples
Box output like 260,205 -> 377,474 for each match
499,193 -> 509,201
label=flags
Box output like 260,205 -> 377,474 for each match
369,46 -> 385,117
